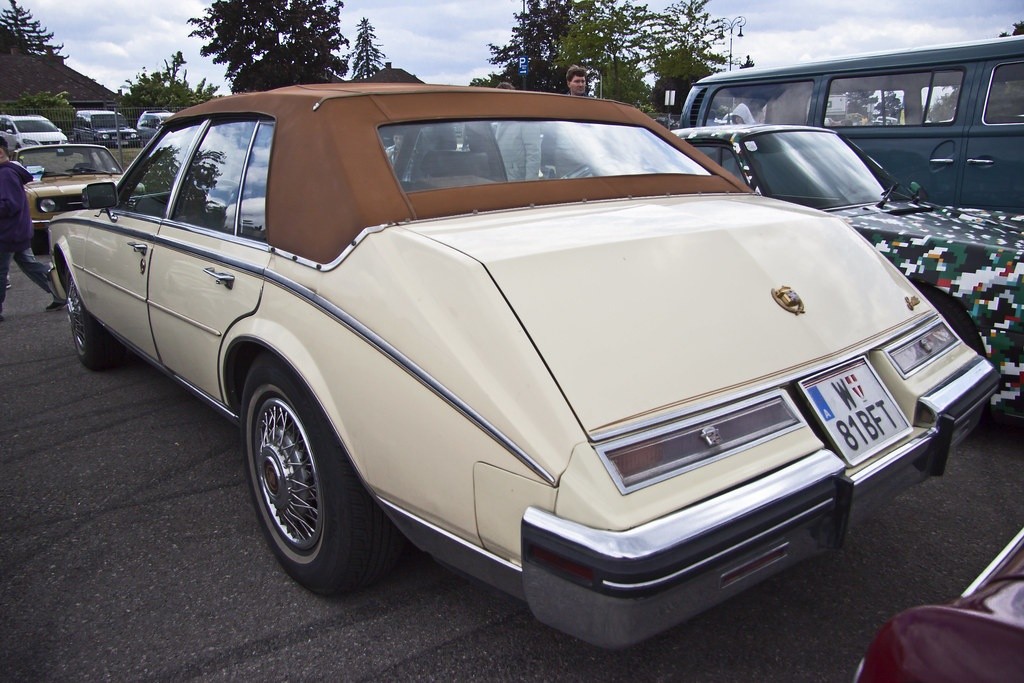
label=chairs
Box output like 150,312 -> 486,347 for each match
413,150 -> 490,193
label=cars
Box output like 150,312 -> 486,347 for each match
673,123 -> 1024,419
45,81 -> 996,642
137,110 -> 175,144
0,113 -> 68,158
13,144 -> 146,257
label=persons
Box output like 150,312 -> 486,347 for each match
492,84 -> 541,188
733,102 -> 754,127
0,135 -> 65,323
562,64 -> 590,99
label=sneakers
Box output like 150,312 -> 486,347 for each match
45,300 -> 68,312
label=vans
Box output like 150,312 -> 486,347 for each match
682,36 -> 1023,216
73,110 -> 141,148
